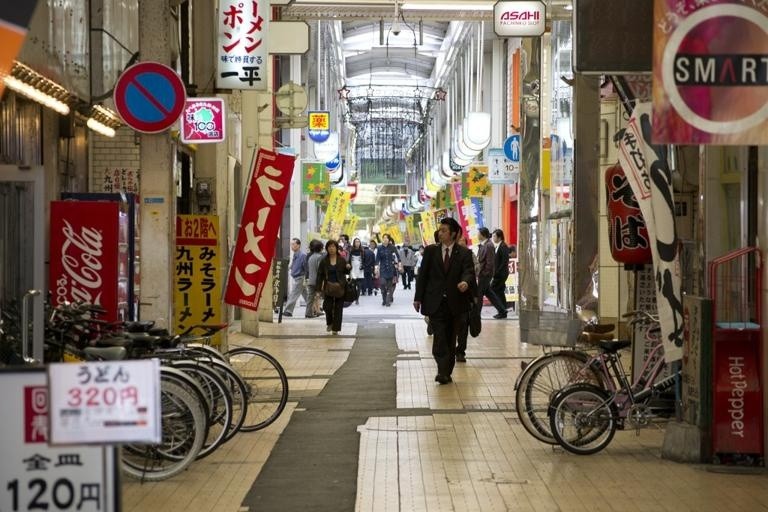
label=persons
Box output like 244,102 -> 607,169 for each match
280,216 -> 517,384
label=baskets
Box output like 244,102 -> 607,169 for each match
518,310 -> 576,346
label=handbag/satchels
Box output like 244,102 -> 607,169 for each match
344,280 -> 360,302
326,281 -> 344,298
470,304 -> 481,337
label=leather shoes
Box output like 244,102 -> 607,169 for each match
494,308 -> 508,319
456,347 -> 466,362
436,374 -> 452,384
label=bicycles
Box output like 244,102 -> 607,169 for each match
510,306 -> 683,458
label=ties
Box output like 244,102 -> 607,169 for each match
444,248 -> 450,274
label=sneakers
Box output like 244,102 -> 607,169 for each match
327,324 -> 333,331
282,311 -> 293,316
332,331 -> 337,335
306,308 -> 324,317
